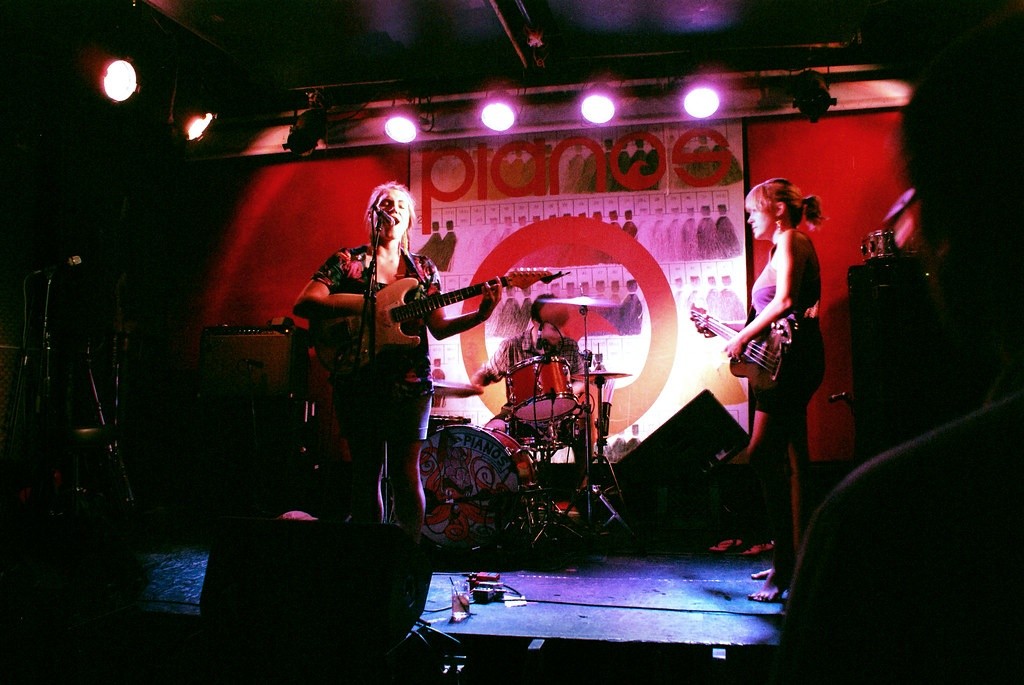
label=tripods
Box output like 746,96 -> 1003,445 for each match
506,305 -> 654,564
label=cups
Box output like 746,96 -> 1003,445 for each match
452,580 -> 470,620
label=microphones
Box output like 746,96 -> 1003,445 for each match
827,392 -> 848,403
536,324 -> 542,350
32,256 -> 82,275
373,205 -> 395,227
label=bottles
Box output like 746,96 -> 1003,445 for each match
431,357 -> 447,409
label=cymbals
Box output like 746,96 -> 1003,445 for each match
571,371 -> 633,381
433,382 -> 484,397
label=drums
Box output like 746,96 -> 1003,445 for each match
428,415 -> 472,435
507,354 -> 580,422
414,424 -> 536,553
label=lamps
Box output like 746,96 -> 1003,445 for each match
287,111 -> 320,155
581,84 -> 614,124
684,74 -> 719,117
792,71 -> 838,121
79,50 -> 140,104
385,105 -> 417,143
482,91 -> 515,132
171,100 -> 216,141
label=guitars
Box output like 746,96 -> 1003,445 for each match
689,302 -> 800,392
308,263 -> 574,377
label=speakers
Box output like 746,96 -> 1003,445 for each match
199,516 -> 434,660
198,327 -> 311,401
0,496 -> 148,644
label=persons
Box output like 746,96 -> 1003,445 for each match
468,293 -> 606,514
291,179 -> 502,542
691,178 -> 826,602
